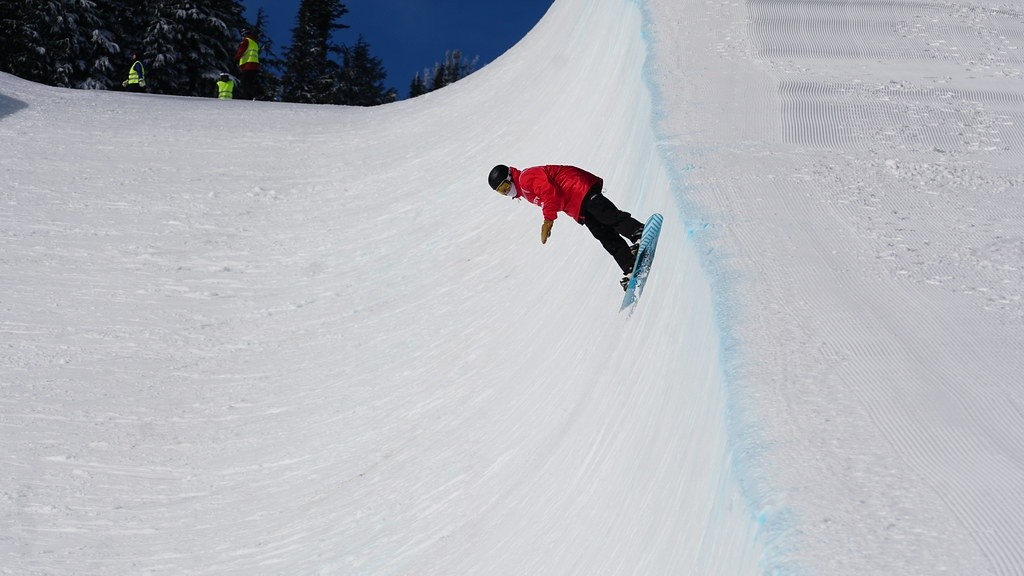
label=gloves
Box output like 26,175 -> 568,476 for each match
541,220 -> 554,244
231,59 -> 236,69
122,79 -> 129,88
138,80 -> 146,88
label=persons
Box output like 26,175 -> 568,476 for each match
234,28 -> 260,100
488,165 -> 646,292
213,70 -> 235,99
122,50 -> 146,92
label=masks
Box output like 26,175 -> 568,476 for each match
507,184 -> 517,198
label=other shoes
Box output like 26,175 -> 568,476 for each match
620,266 -> 635,292
630,222 -> 646,243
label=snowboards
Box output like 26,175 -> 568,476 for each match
618,213 -> 664,314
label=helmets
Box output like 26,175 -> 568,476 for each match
240,27 -> 250,36
487,164 -> 509,190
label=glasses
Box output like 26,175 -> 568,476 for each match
497,180 -> 511,195
